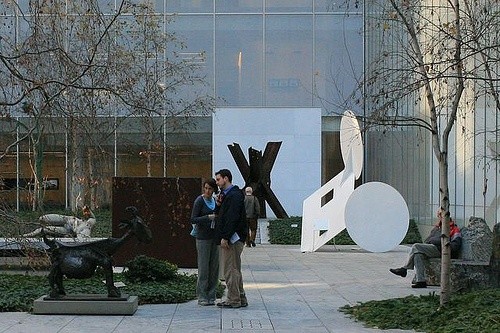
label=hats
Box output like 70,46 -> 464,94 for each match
245,187 -> 252,195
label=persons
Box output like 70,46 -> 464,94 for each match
190,177 -> 220,306
244,186 -> 261,247
389,206 -> 463,288
214,169 -> 248,308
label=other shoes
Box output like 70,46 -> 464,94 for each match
198,299 -> 216,306
245,245 -> 251,247
389,268 -> 407,278
412,281 -> 427,288
217,300 -> 241,308
241,302 -> 248,307
250,240 -> 256,247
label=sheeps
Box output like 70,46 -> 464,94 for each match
42,205 -> 153,298
